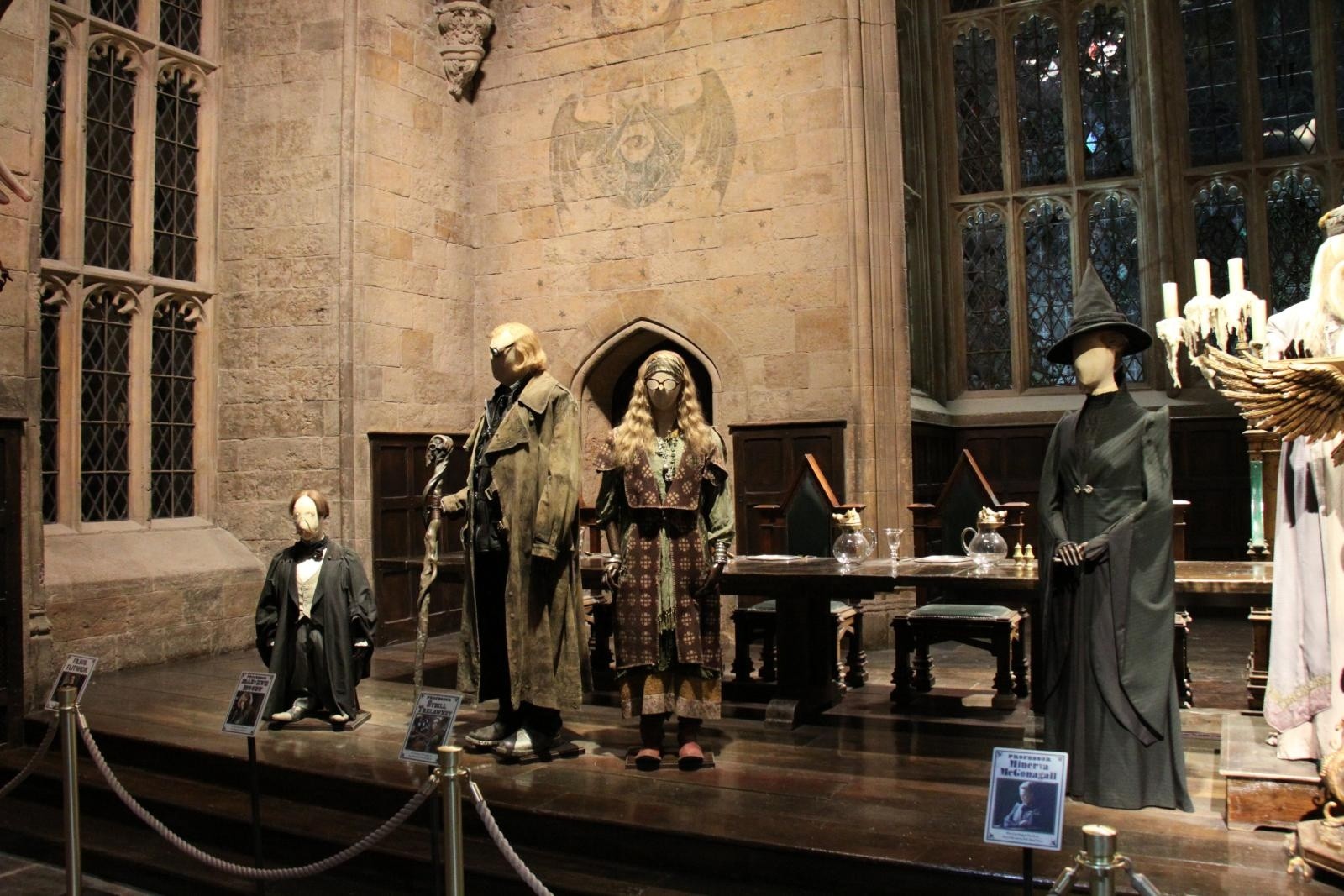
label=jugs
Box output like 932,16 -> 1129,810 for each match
959,525 -> 1008,565
833,523 -> 876,564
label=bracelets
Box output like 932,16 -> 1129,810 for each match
603,557 -> 622,564
714,541 -> 728,564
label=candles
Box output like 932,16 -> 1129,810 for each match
1160,279 -> 1179,319
1227,258 -> 1245,293
1194,258 -> 1212,297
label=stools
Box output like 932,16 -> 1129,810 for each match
891,600 -> 1030,710
729,597 -> 867,693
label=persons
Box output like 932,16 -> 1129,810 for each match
1003,781 -> 1042,832
1033,323 -> 1197,813
255,489 -> 379,722
1258,232 -> 1344,737
593,349 -> 737,770
440,320 -> 595,756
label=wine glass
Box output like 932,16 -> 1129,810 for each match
884,527 -> 903,557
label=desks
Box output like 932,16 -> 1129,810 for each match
711,548 -> 1276,728
